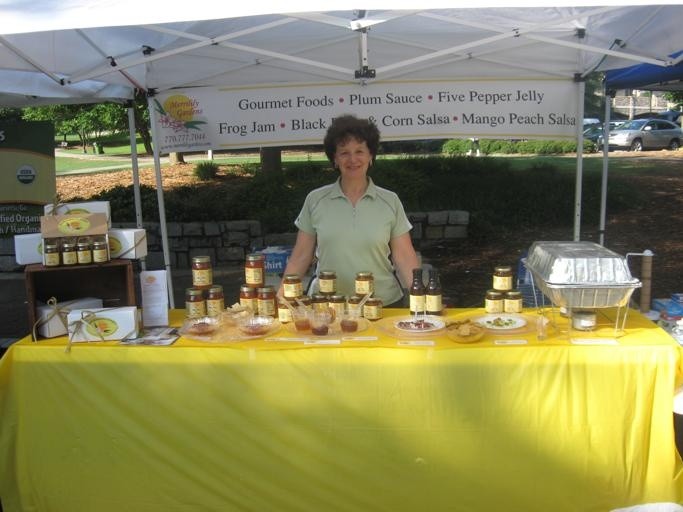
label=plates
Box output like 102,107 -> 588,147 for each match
479,314 -> 525,329
395,314 -> 443,335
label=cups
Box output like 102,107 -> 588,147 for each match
293,308 -> 357,334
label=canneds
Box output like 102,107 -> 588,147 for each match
45,242 -> 108,267
484,266 -> 523,313
185,256 -> 224,319
239,254 -> 384,324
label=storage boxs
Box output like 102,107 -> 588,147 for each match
44,200 -> 113,228
38,214 -> 108,267
36,294 -> 104,341
137,266 -> 172,331
18,254 -> 135,345
107,227 -> 148,260
250,247 -> 316,290
12,233 -> 46,265
66,305 -> 139,343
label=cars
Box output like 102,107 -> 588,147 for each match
581,109 -> 683,151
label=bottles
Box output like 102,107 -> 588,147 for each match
409,268 -> 425,314
427,270 -> 442,314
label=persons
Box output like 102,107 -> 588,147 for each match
274,114 -> 421,307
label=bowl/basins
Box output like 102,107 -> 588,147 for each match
444,317 -> 486,343
238,315 -> 272,335
182,315 -> 222,333
